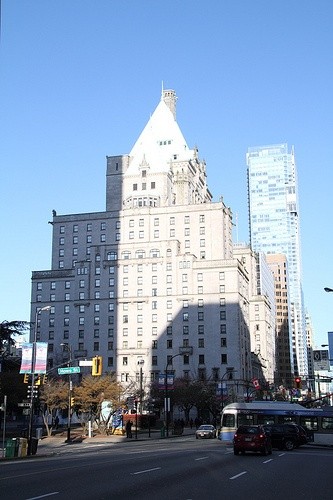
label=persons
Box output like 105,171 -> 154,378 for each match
173,417 -> 217,430
54,416 -> 60,429
125,420 -> 134,439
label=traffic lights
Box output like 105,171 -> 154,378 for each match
296,378 -> 301,389
27,385 -> 31,398
33,385 -> 39,398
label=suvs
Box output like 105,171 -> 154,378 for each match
263,422 -> 309,451
233,425 -> 273,456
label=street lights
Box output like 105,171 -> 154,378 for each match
28,306 -> 52,455
221,370 -> 239,410
60,343 -> 73,443
164,350 -> 191,439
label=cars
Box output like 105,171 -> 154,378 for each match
195,425 -> 217,439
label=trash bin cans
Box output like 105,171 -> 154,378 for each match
6,437 -> 27,457
36,428 -> 44,438
161,425 -> 165,436
28,437 -> 38,455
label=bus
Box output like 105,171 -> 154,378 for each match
220,400 -> 333,447
